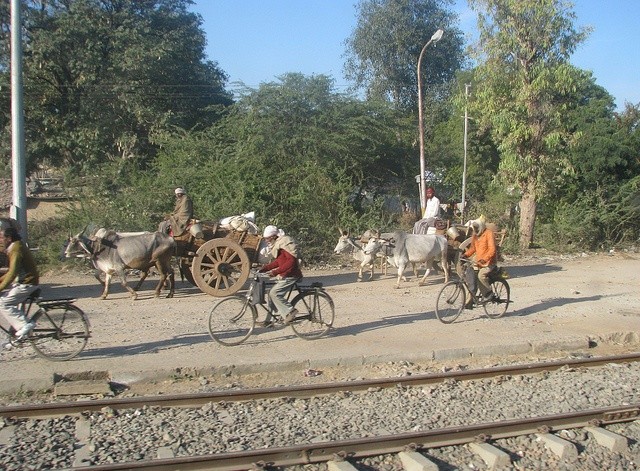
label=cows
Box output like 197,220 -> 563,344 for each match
334,225 -> 422,282
362,230 -> 449,289
60,227 -> 169,289
64,224 -> 184,300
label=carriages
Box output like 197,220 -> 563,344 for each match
64,220 -> 263,300
334,226 -> 507,289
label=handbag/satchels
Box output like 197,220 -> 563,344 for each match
251,274 -> 267,306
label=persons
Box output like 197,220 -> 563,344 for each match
159,187 -> 191,235
461,218 -> 496,307
413,188 -> 440,234
0,219 -> 38,348
254,225 -> 302,324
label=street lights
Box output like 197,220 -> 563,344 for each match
417,30 -> 444,219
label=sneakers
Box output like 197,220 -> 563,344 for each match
3,342 -> 23,350
284,309 -> 299,322
15,322 -> 36,336
481,292 -> 495,303
464,298 -> 475,309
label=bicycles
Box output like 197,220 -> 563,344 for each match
435,255 -> 510,324
0,288 -> 92,361
207,269 -> 335,346
